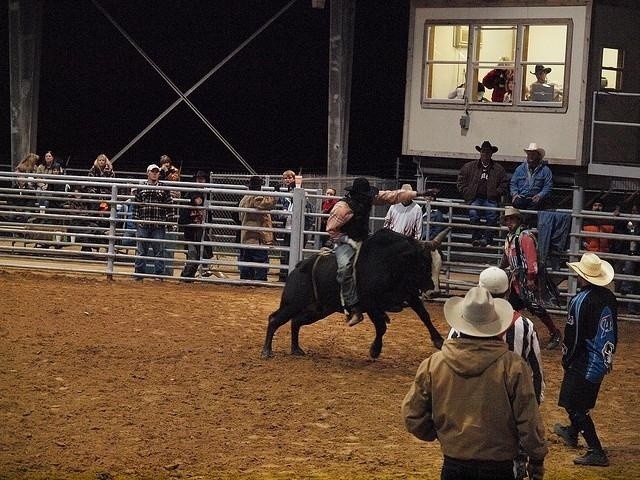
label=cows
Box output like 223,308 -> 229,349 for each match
259,226 -> 452,361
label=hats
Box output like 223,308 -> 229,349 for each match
147,164 -> 160,172
245,176 -> 264,187
444,267 -> 513,337
566,253 -> 614,286
530,64 -> 551,74
344,177 -> 378,197
476,141 -> 498,153
500,208 -> 522,224
524,143 -> 545,159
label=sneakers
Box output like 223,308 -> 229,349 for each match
349,313 -> 363,326
574,451 -> 608,466
554,423 -> 577,446
547,329 -> 561,349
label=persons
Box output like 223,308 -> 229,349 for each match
483,55 -> 513,101
497,203 -> 561,353
231,176 -> 279,287
383,184 -> 423,308
399,286 -> 549,480
525,64 -> 562,101
68,186 -> 138,252
581,200 -> 639,315
422,187 -> 450,299
457,141 -> 508,246
87,154 -> 116,200
552,251 -> 618,466
9,151 -> 74,249
276,169 -> 308,282
449,74 -> 490,102
327,177 -> 440,325
503,75 -> 514,103
510,142 -> 555,210
320,188 -> 338,246
448,264 -> 545,480
132,155 -> 216,282
